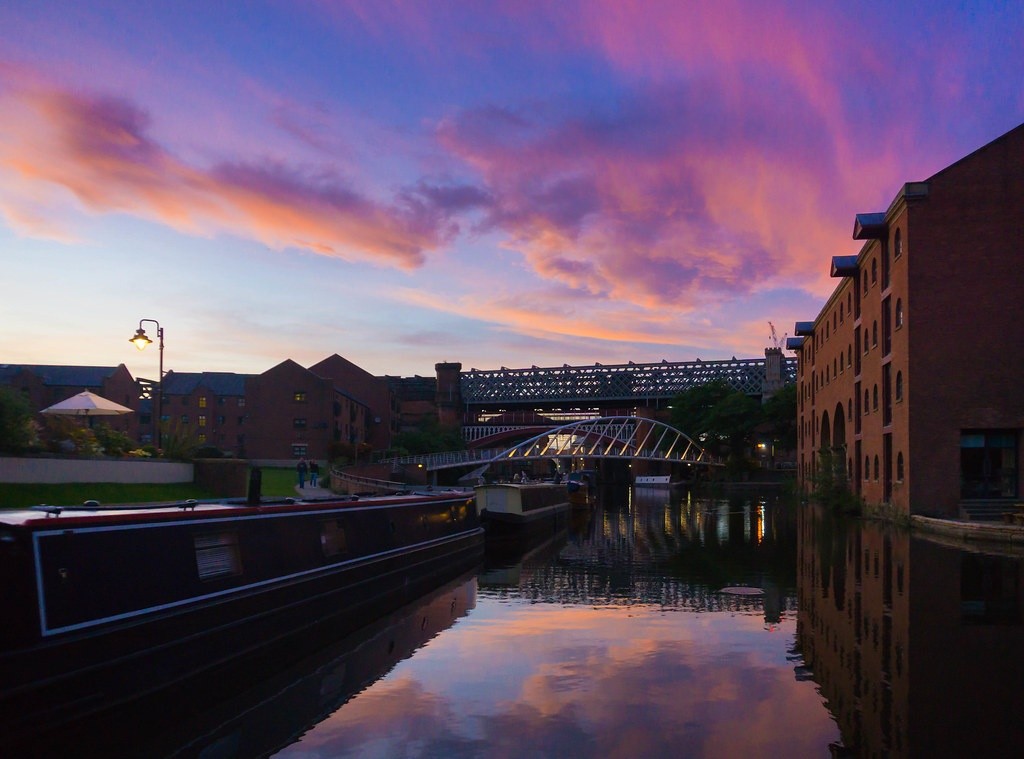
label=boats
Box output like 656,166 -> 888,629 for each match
475,480 -> 574,538
0,489 -> 486,759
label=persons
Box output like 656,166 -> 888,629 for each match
498,471 -> 568,484
297,457 -> 308,489
309,457 -> 318,487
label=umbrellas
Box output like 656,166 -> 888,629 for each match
38,387 -> 134,430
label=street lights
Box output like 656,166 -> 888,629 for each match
129,319 -> 170,447
